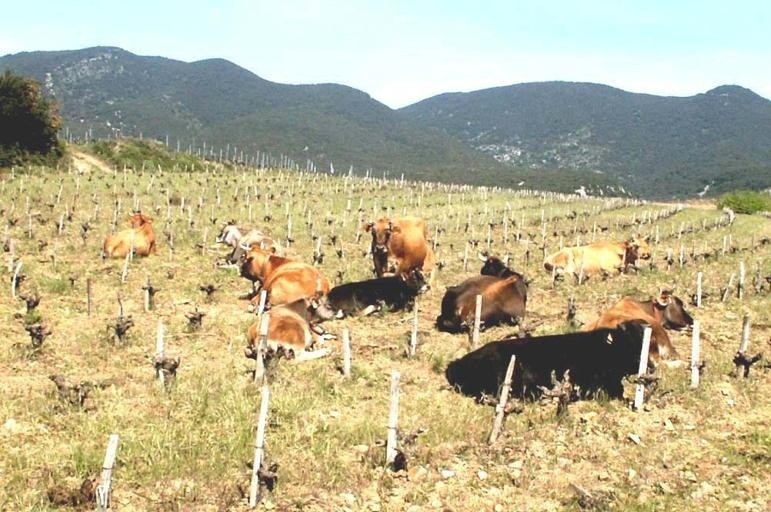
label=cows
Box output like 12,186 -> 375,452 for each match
586,279 -> 696,371
235,241 -> 330,312
436,249 -> 529,333
214,214 -> 283,271
445,318 -> 661,408
362,215 -> 429,279
243,291 -> 345,363
102,210 -> 157,258
326,265 -> 432,320
542,233 -> 653,284
